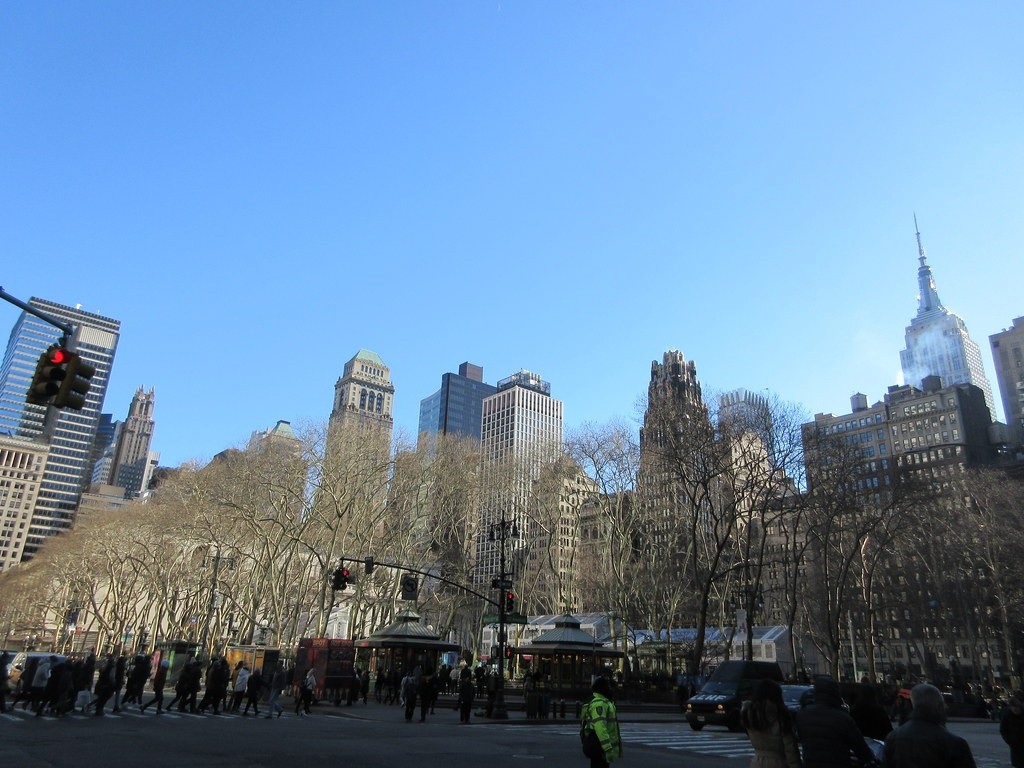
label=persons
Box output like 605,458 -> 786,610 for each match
0,647 -> 553,724
740,673 -> 1023,768
579,678 -> 624,768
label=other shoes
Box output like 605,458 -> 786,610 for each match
242,712 -> 250,717
305,711 -> 313,715
93,712 -> 105,718
430,712 -> 436,715
363,699 -> 367,704
419,719 -> 426,723
264,716 -> 273,719
177,707 -> 205,715
8,705 -> 16,712
223,709 -> 241,714
212,712 -> 221,715
277,709 -> 283,719
112,706 -> 122,713
157,710 -> 165,715
400,702 -> 405,708
21,704 -> 27,711
139,705 -> 145,715
82,704 -> 89,713
401,719 -> 412,723
166,707 -> 172,712
254,711 -> 261,718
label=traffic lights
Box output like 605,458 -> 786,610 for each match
507,593 -> 514,610
25,346 -> 73,405
55,356 -> 96,411
505,648 -> 514,658
337,568 -> 349,590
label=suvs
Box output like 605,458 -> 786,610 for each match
685,660 -> 784,733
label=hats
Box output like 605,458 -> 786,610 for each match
161,660 -> 169,668
189,657 -> 197,664
910,685 -> 945,712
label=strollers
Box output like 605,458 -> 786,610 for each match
851,736 -> 886,768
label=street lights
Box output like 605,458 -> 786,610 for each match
486,508 -> 518,719
198,544 -> 236,657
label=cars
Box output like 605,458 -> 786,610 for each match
781,683 -> 814,715
7,650 -> 66,696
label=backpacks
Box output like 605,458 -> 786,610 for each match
300,676 -> 312,690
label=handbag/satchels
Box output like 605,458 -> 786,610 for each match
579,729 -> 607,760
